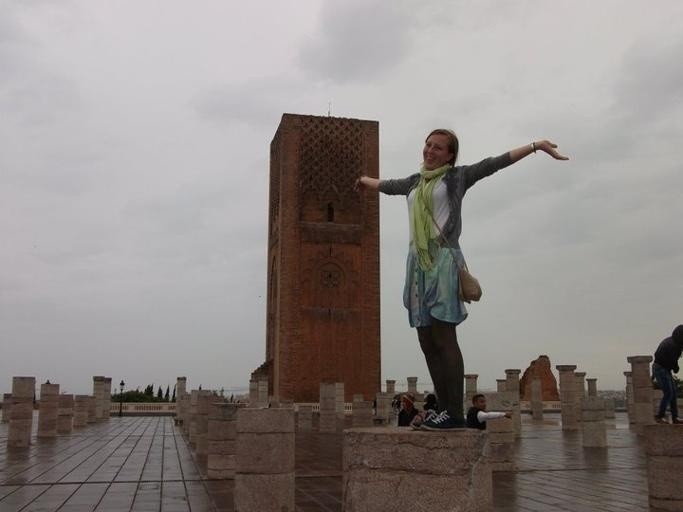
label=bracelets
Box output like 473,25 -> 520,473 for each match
531,140 -> 538,154
357,175 -> 367,186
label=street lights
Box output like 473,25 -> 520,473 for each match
119,380 -> 125,416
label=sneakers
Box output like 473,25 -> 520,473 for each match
410,409 -> 438,430
673,418 -> 683,423
422,410 -> 465,431
657,418 -> 669,424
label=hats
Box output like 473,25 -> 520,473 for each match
402,393 -> 415,411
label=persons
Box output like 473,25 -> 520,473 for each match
391,393 -> 438,427
351,128 -> 570,431
651,324 -> 683,424
467,395 -> 512,429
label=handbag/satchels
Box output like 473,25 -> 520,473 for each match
458,270 -> 481,302
